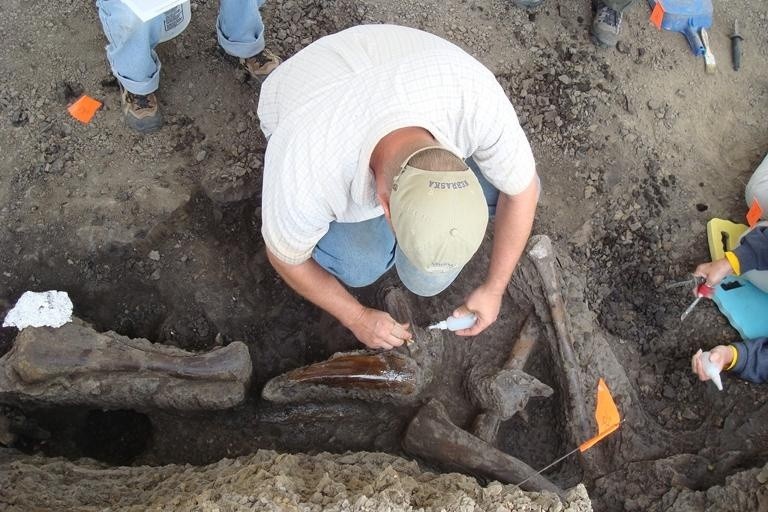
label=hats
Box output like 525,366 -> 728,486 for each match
389,147 -> 489,298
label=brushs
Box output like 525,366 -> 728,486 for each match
700,27 -> 716,73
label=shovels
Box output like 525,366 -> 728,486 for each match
648,0 -> 714,55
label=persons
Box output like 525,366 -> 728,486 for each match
94,0 -> 284,132
257,24 -> 542,352
513,0 -> 635,47
691,153 -> 768,381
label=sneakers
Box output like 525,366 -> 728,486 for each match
117,79 -> 162,131
220,45 -> 282,84
592,0 -> 622,47
